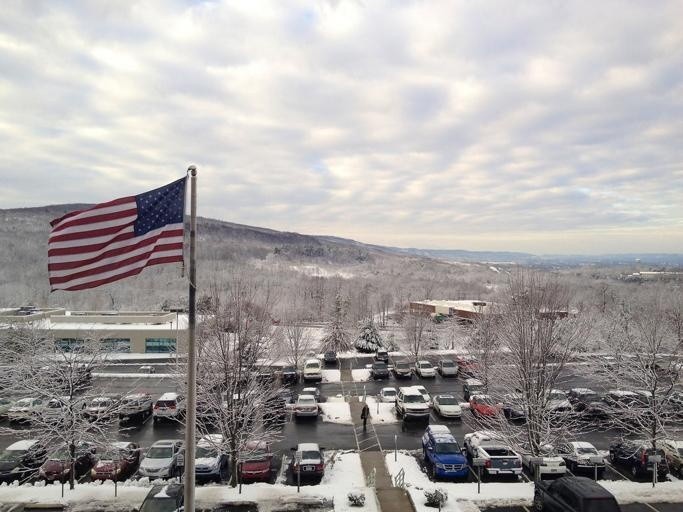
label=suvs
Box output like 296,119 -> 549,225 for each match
376,348 -> 388,362
421,423 -> 469,480
534,476 -> 619,511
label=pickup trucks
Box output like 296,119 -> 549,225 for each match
464,431 -> 522,481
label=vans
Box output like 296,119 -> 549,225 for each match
609,438 -> 671,480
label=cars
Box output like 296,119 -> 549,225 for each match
371,360 -> 387,378
655,439 -> 683,476
139,484 -> 184,512
324,350 -> 337,364
438,359 -> 457,377
393,361 -> 411,379
515,440 -> 567,476
414,360 -> 435,377
558,440 -> 606,474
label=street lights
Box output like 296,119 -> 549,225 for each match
170,308 -> 185,370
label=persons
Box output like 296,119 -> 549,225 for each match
360,405 -> 369,432
462,382 -> 467,398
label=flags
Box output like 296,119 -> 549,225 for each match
48,174 -> 188,293
